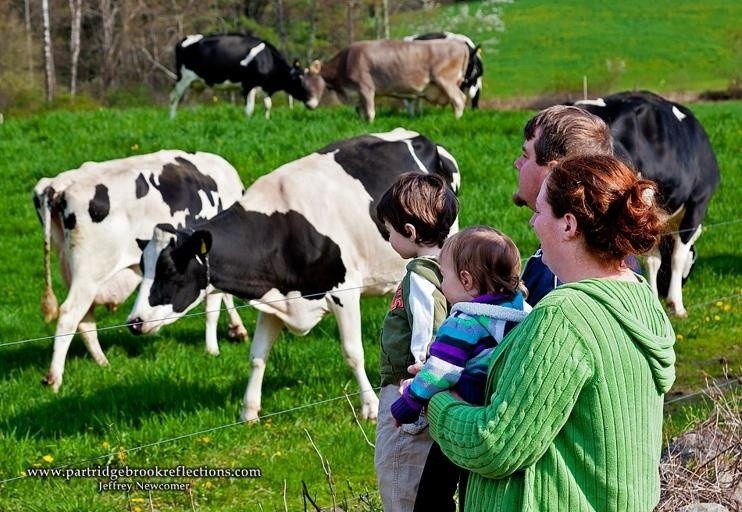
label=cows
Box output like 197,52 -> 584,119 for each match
561,88 -> 722,321
125,125 -> 463,428
302,38 -> 471,124
31,148 -> 250,395
402,30 -> 484,118
167,32 -> 314,121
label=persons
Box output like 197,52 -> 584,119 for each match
375,173 -> 461,511
406,154 -> 677,512
513,106 -> 643,302
391,226 -> 534,512
